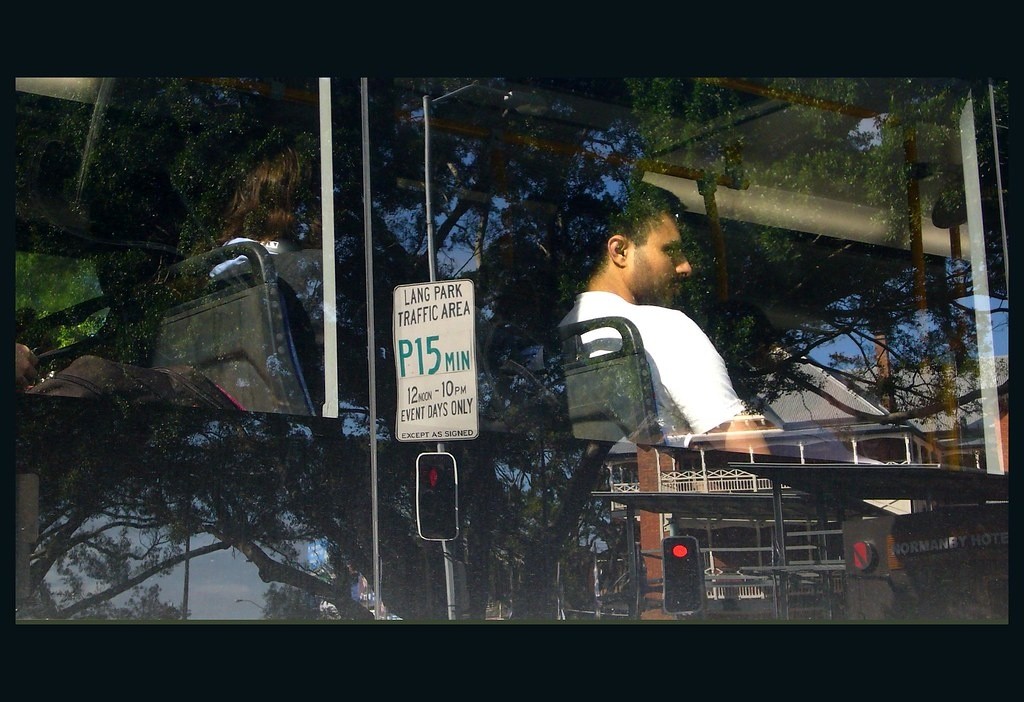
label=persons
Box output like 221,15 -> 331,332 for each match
557,179 -> 773,460
167,137 -> 368,413
14,341 -> 41,387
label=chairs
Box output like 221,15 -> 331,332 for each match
492,316 -> 666,448
127,242 -> 317,418
18,288 -> 127,418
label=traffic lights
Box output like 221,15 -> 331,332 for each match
659,535 -> 706,616
415,451 -> 459,542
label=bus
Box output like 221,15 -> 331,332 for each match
0,0 -> 1023,702
